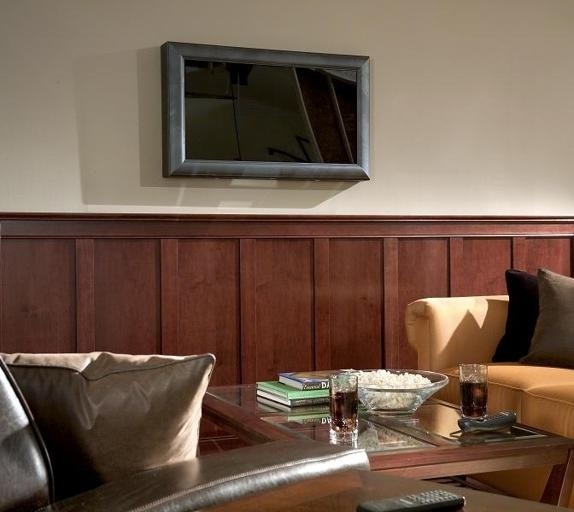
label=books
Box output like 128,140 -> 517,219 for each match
256,396 -> 369,425
254,368 -> 365,408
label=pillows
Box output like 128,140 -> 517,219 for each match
491,264 -> 574,361
0,345 -> 219,497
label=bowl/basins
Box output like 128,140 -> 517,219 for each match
334,368 -> 450,414
355,419 -> 432,454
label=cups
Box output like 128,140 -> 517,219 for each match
328,372 -> 359,442
459,362 -> 488,421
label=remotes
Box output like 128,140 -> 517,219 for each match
356,489 -> 465,512
459,424 -> 512,444
458,410 -> 516,433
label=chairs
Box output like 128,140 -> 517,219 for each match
0,338 -> 374,512
405,289 -> 574,507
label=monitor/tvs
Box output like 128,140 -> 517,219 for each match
160,41 -> 370,181
